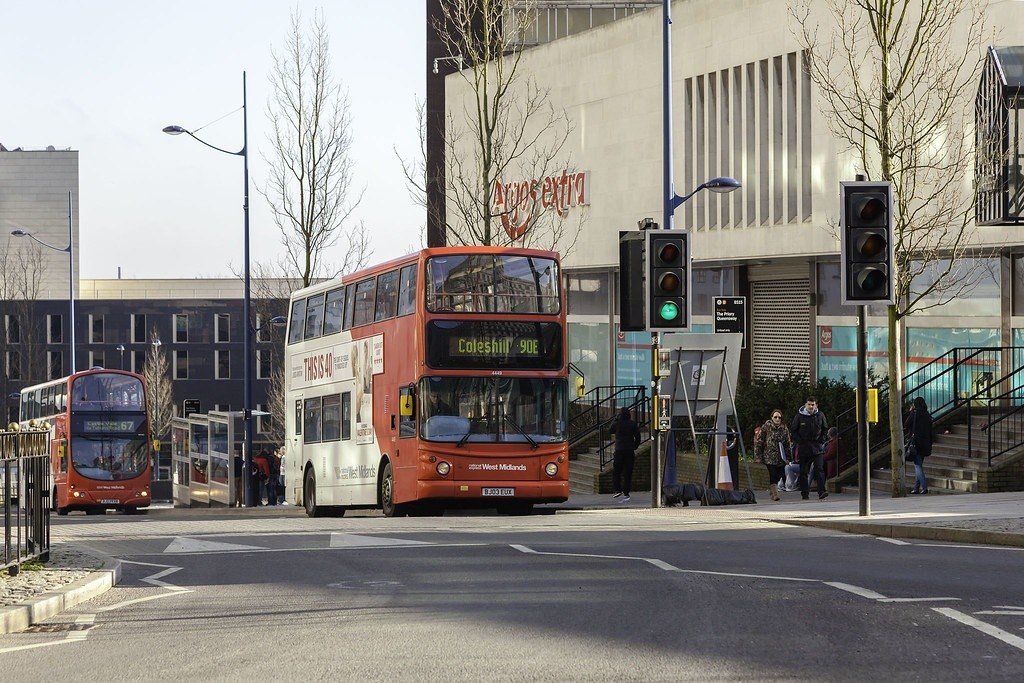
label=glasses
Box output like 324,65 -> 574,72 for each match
773,416 -> 781,419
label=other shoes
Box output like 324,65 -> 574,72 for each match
819,492 -> 828,498
613,491 -> 621,497
920,488 -> 927,494
623,495 -> 630,499
911,490 -> 919,493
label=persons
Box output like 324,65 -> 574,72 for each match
62,406 -> 67,412
606,407 -> 642,498
778,427 -> 843,492
756,408 -> 792,500
54,405 -> 59,414
255,445 -> 286,505
904,396 -> 935,494
782,395 -> 829,501
424,388 -> 452,417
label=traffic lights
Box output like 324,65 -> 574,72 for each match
646,229 -> 693,333
839,182 -> 896,305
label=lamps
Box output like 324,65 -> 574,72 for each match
807,293 -> 815,306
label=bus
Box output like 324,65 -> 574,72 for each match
284,247 -> 585,518
18,367 -> 161,516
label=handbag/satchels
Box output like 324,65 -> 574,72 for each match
904,436 -> 916,461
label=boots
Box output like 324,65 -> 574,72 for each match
770,484 -> 780,501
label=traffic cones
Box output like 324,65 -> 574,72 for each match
718,441 -> 734,492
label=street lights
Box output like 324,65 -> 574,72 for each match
662,0 -> 742,230
163,71 -> 260,508
11,192 -> 77,376
